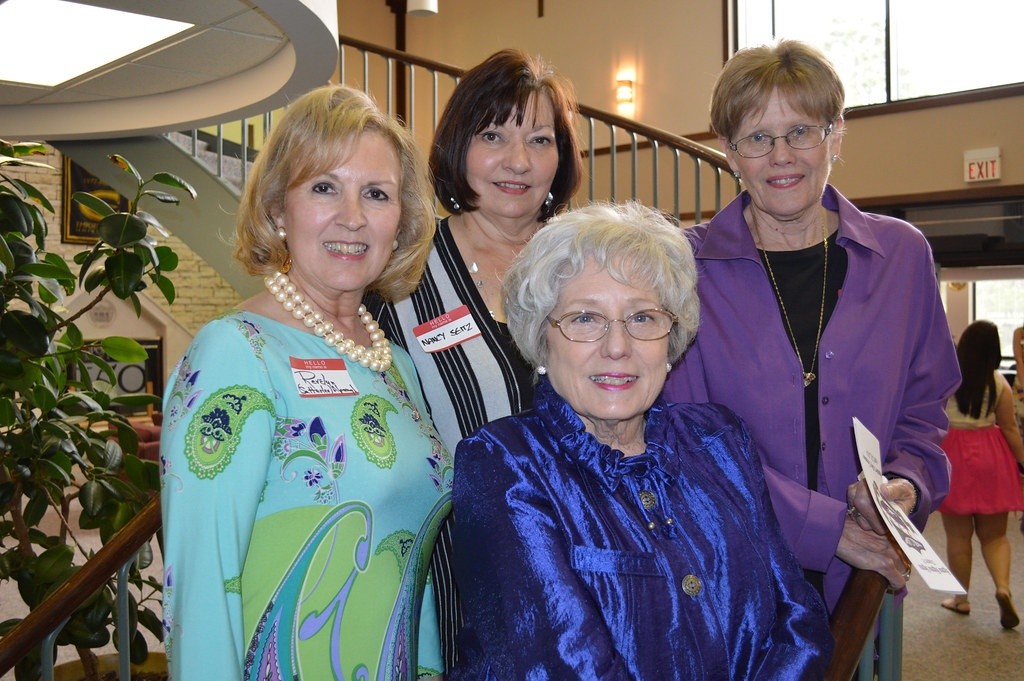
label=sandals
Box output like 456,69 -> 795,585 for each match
995,585 -> 1020,629
939,598 -> 972,615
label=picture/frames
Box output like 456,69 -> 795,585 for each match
60,154 -> 136,244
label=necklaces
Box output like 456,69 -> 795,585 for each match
749,203 -> 827,387
264,269 -> 392,372
460,214 -> 514,338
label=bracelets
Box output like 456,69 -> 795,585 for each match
909,480 -> 918,515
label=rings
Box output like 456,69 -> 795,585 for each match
902,567 -> 911,576
847,507 -> 860,520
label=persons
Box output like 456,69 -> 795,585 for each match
361,47 -> 585,673
933,320 -> 1024,629
1012,324 -> 1024,535
449,199 -> 835,681
160,85 -> 451,681
654,40 -> 962,681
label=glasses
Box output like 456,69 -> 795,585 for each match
543,310 -> 679,341
726,124 -> 836,158
